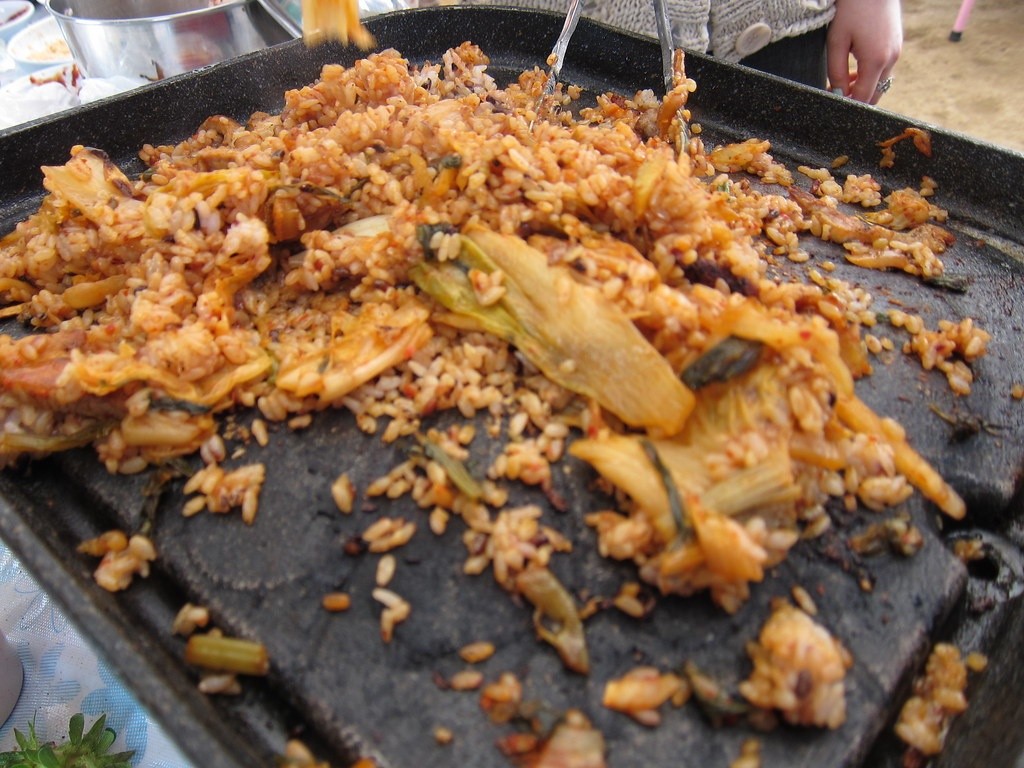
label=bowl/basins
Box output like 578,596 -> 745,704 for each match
45,0 -> 254,82
0,0 -> 94,138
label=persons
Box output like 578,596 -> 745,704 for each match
454,0 -> 901,105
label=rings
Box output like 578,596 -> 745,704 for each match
876,77 -> 893,92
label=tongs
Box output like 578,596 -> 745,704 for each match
525,1 -> 694,160
